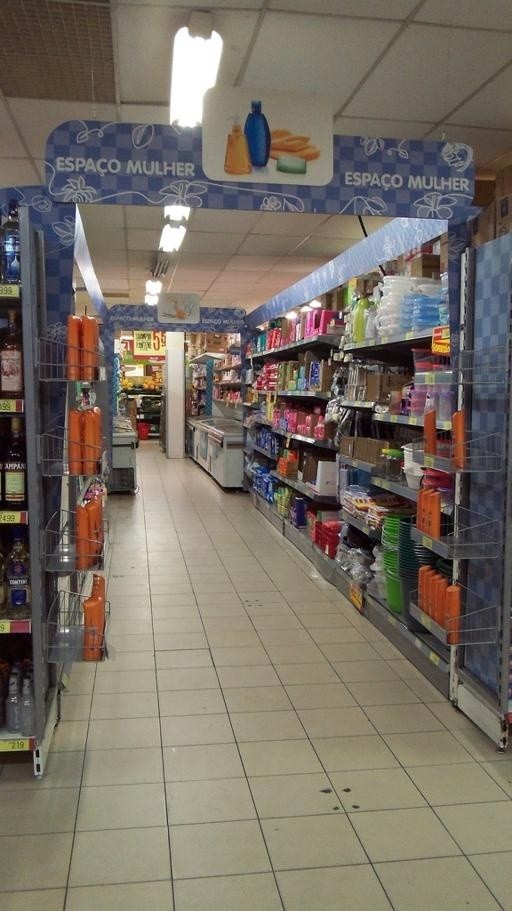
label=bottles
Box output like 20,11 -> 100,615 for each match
244,101 -> 271,167
0,199 -> 33,738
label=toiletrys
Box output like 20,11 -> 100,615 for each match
66,315 -> 105,660
419,393 -> 465,644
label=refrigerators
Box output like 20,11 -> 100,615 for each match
109,421 -> 138,496
186,417 -> 245,490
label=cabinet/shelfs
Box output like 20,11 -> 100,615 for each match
0,280 -> 463,782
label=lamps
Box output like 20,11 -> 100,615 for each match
142,8 -> 224,308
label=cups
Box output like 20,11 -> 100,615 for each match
277,155 -> 306,174
